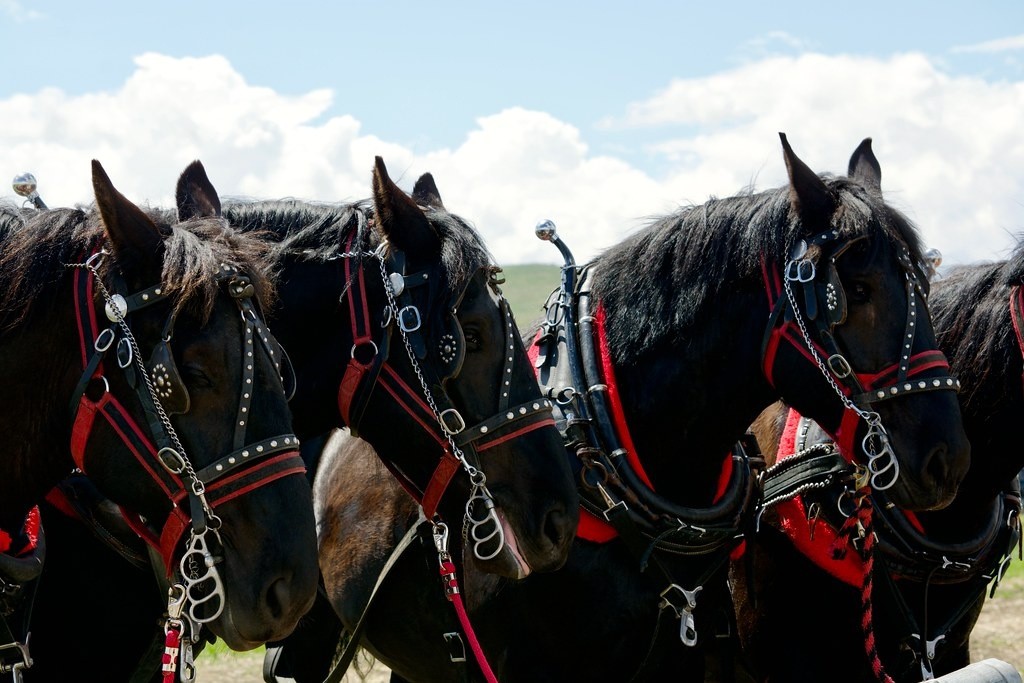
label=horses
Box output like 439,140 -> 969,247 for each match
312,132 -> 1024,682
0,156 -> 578,683
0,159 -> 319,683
